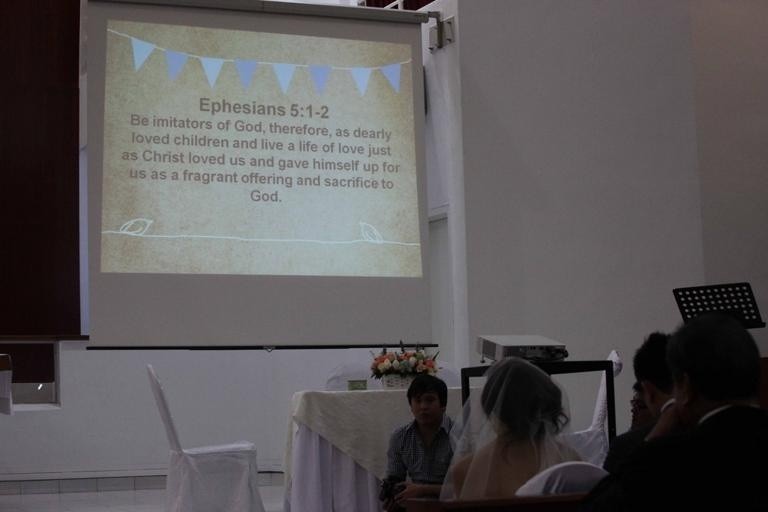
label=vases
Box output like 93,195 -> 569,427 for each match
381,373 -> 416,389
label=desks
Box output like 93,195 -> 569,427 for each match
289,384 -> 570,509
461,362 -> 617,460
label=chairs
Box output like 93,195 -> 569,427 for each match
146,363 -> 263,512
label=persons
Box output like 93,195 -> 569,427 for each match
584,312 -> 768,512
452,350 -> 623,503
383,374 -> 461,512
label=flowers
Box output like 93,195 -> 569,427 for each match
368,341 -> 443,381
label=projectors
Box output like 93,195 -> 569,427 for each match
475,334 -> 566,362
